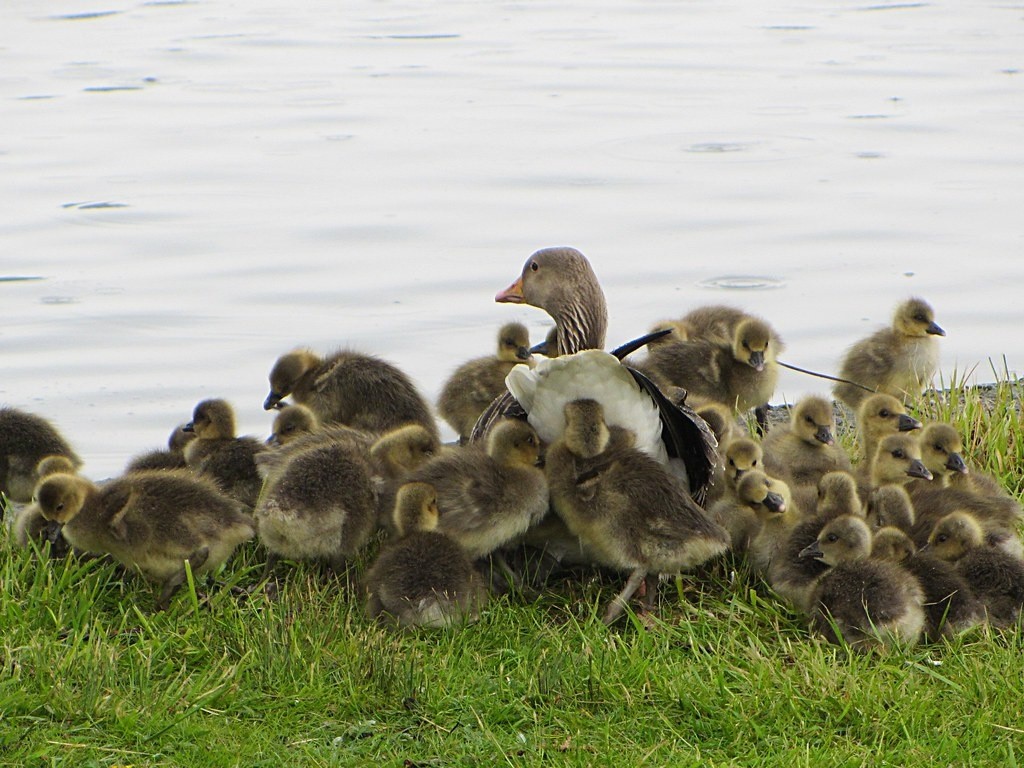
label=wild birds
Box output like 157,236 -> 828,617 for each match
834,299 -> 945,415
0,393 -> 269,561
31,469 -> 257,644
466,247 -> 717,626
715,394 -> 1024,651
261,323 -> 534,633
698,297 -> 782,436
